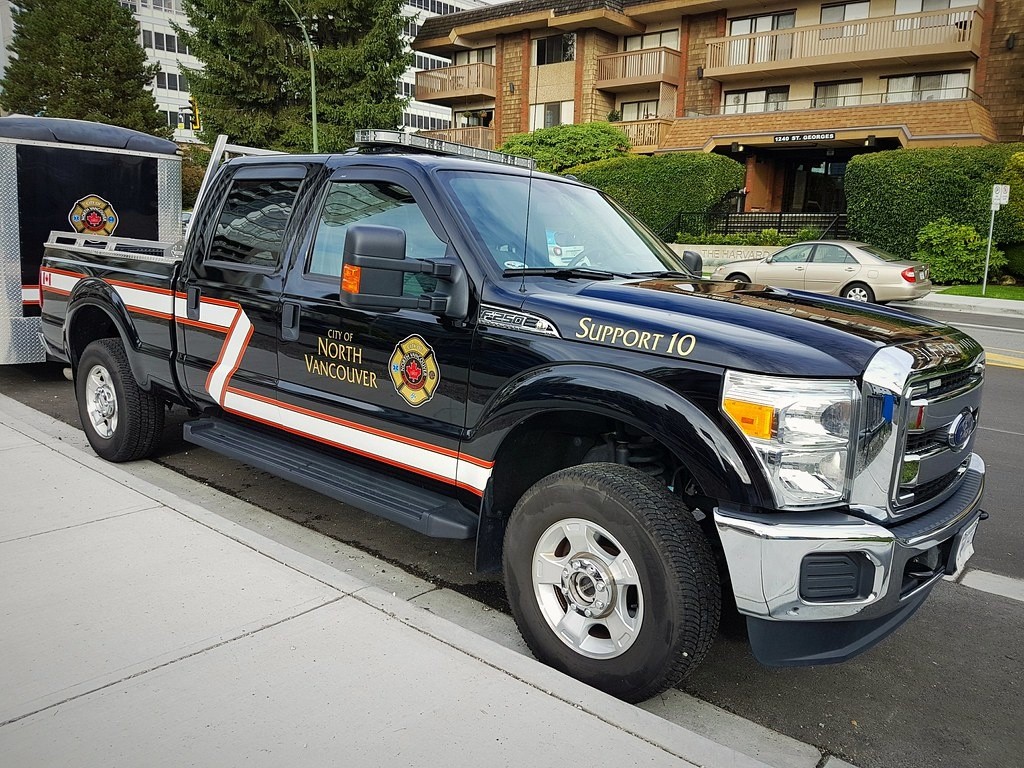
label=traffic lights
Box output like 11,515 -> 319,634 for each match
188,95 -> 200,130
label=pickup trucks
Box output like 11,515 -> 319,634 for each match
31,125 -> 987,704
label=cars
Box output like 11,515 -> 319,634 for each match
710,240 -> 932,305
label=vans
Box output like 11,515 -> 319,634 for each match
0,115 -> 186,368
